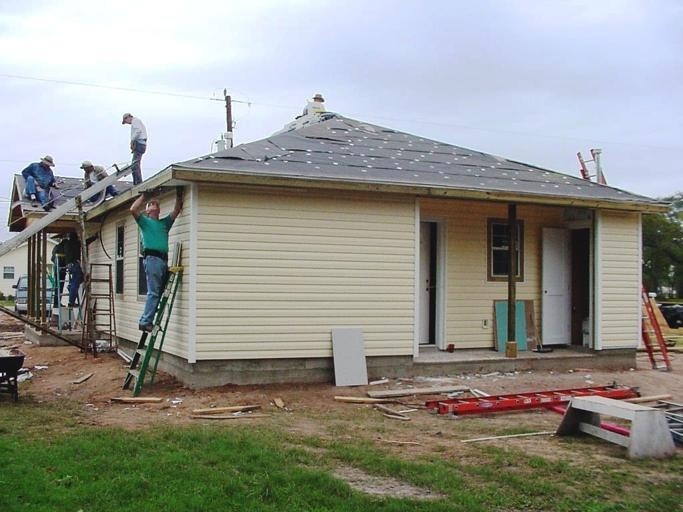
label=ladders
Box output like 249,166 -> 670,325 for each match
122,265 -> 184,398
80,263 -> 117,359
48,253 -> 85,336
424,384 -> 641,415
576,149 -> 673,370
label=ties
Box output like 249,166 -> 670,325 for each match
40,155 -> 56,167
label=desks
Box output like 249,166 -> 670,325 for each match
0,348 -> 25,402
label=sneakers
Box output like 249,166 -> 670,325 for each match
67,301 -> 79,308
30,197 -> 39,207
138,324 -> 163,331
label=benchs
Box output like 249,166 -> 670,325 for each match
551,394 -> 676,460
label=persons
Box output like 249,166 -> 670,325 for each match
295,92 -> 325,121
63,231 -> 101,308
129,184 -> 182,332
49,239 -> 70,309
80,160 -> 120,201
22,155 -> 59,211
121,112 -> 148,185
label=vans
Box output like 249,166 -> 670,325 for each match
13,276 -> 55,314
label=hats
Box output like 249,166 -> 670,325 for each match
121,112 -> 133,124
312,94 -> 324,102
79,160 -> 93,169
